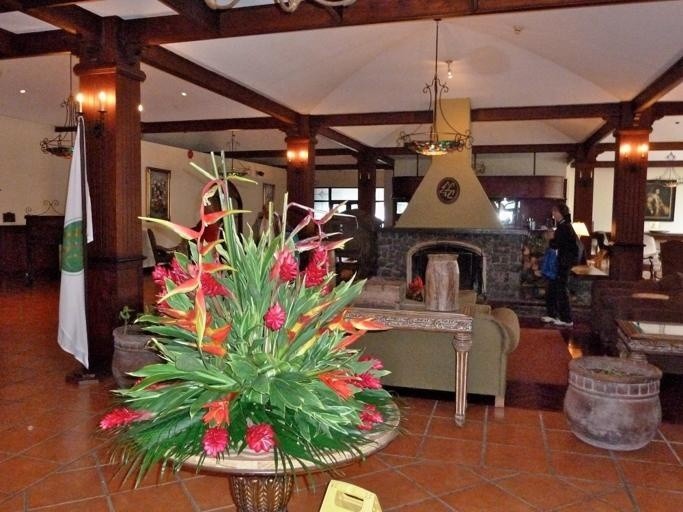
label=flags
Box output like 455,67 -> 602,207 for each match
54,117 -> 96,373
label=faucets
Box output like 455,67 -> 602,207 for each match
658,322 -> 665,335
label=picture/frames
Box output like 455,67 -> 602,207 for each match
144,166 -> 171,222
643,180 -> 677,221
262,183 -> 275,209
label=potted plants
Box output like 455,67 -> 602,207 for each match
111,305 -> 167,389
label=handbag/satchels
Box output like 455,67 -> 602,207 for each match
541,248 -> 558,280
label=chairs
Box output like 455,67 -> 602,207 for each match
590,272 -> 683,348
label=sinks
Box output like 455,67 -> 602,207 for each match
629,320 -> 683,336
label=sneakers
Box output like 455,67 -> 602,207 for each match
540,316 -> 574,327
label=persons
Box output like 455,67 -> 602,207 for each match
540,201 -> 575,329
646,188 -> 669,217
251,203 -> 281,248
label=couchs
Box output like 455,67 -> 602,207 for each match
345,302 -> 521,408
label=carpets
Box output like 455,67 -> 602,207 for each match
502,317 -> 682,424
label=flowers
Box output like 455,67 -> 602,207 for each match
84,146 -> 409,498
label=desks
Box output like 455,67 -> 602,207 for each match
126,398 -> 401,512
344,308 -> 472,428
571,264 -> 609,288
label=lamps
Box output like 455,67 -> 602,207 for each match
217,131 -> 247,177
39,52 -> 78,160
654,152 -> 683,188
395,18 -> 475,156
74,90 -> 108,138
